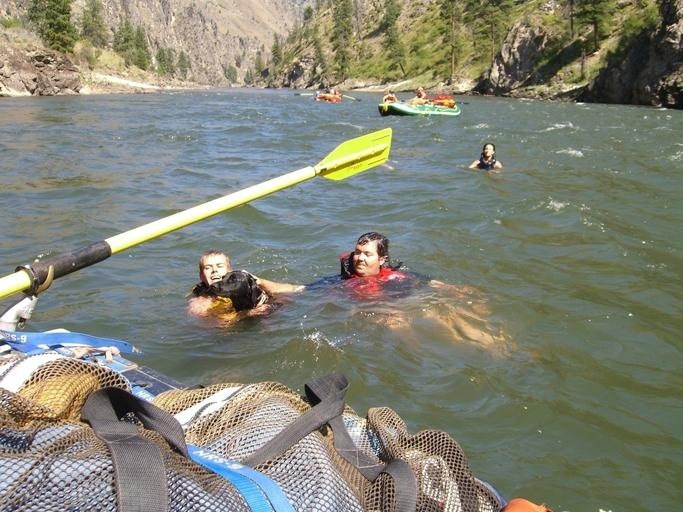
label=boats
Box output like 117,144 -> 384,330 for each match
315,93 -> 343,103
377,102 -> 463,117
1,323 -> 510,512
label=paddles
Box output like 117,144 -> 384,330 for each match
423,100 -> 469,105
338,94 -> 361,103
0,127 -> 392,298
293,93 -> 317,97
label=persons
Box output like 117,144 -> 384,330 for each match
383,90 -> 401,102
184,247 -> 283,330
314,81 -> 339,104
408,88 -> 428,103
238,230 -> 510,360
467,141 -> 504,172
381,88 -> 398,104
